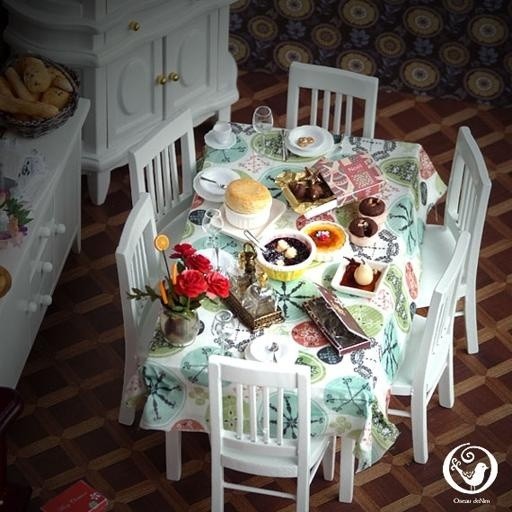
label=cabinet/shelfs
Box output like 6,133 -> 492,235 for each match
106,1 -> 229,154
2,97 -> 92,390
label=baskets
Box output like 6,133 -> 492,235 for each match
1,54 -> 79,138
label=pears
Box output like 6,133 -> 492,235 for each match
354,259 -> 374,286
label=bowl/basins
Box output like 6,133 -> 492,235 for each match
253,228 -> 317,282
300,221 -> 350,262
288,124 -> 326,152
249,334 -> 291,362
223,201 -> 273,230
198,167 -> 236,196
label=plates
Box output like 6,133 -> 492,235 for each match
193,248 -> 238,300
330,255 -> 390,299
284,125 -> 334,158
204,130 -> 237,150
244,337 -> 299,364
210,197 -> 287,244
192,166 -> 241,203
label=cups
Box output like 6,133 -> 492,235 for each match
213,122 -> 232,145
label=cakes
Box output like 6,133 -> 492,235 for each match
349,217 -> 384,247
358,195 -> 388,224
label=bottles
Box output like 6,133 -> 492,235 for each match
241,272 -> 278,319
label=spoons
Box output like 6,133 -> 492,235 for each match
200,176 -> 228,190
215,247 -> 222,273
267,341 -> 279,363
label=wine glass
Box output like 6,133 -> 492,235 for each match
210,309 -> 235,355
252,105 -> 274,159
202,208 -> 225,250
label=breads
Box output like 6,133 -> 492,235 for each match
224,178 -> 271,214
